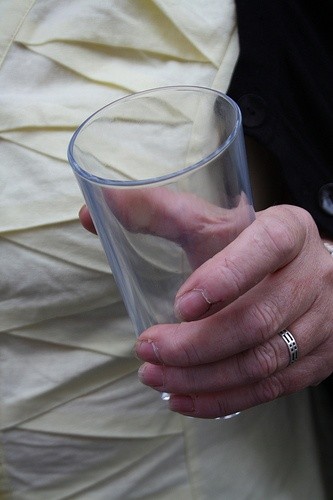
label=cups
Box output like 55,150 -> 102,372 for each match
68,83 -> 259,420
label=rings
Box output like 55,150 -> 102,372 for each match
279,329 -> 299,368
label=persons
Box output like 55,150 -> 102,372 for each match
0,2 -> 333,500
